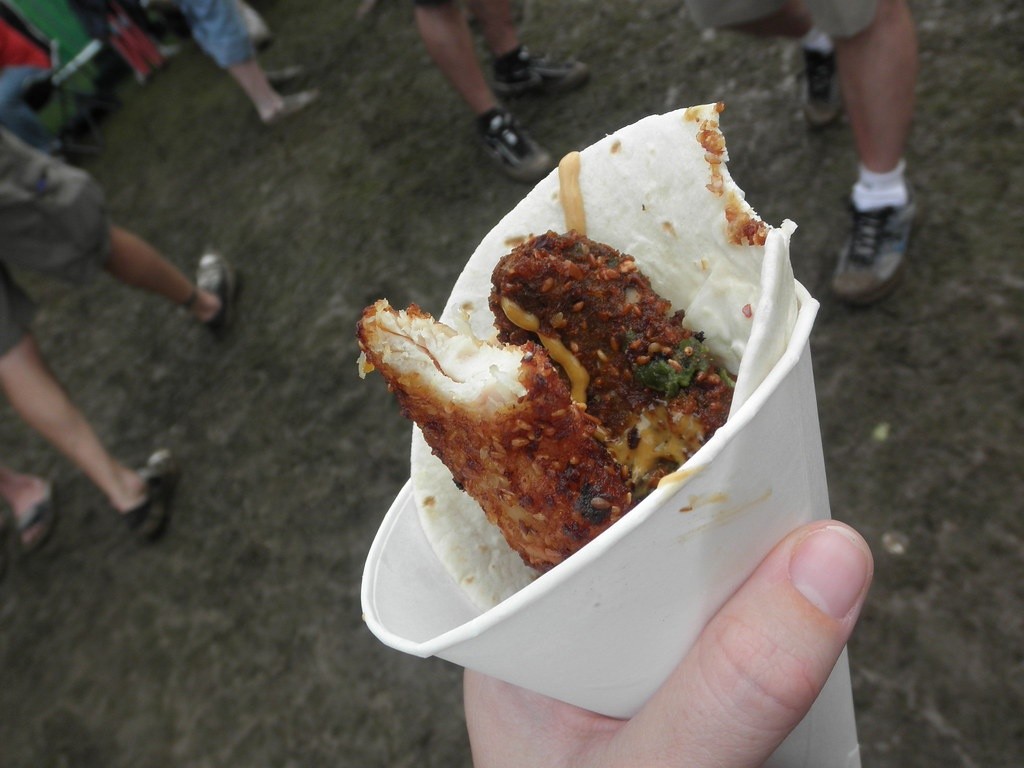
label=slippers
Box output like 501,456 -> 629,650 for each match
18,476 -> 54,557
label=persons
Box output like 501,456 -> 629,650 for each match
179,0 -> 319,123
0,464 -> 55,549
462,520 -> 874,768
0,127 -> 237,537
688,0 -> 915,302
0,21 -> 61,155
414,1 -> 588,182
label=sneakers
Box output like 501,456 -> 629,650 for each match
796,43 -> 844,129
492,51 -> 588,99
474,113 -> 552,180
826,178 -> 916,307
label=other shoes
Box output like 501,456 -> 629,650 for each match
193,250 -> 234,338
267,64 -> 306,86
128,447 -> 180,543
263,88 -> 318,126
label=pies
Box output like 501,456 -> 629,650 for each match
407,102 -> 794,607
